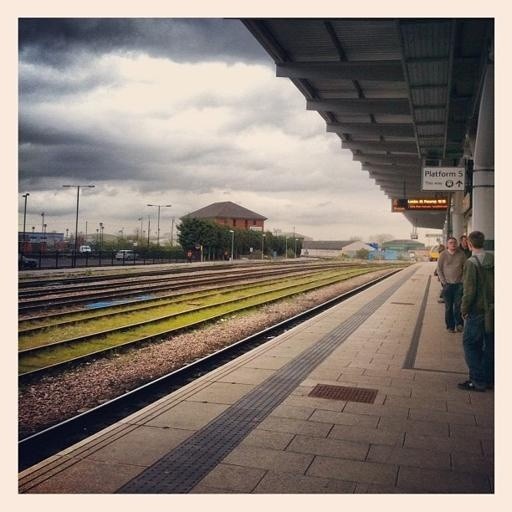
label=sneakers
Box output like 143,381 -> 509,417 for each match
458,381 -> 483,391
449,325 -> 462,333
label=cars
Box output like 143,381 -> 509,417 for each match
18,253 -> 37,271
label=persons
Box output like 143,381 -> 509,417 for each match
187,250 -> 192,263
457,230 -> 494,393
434,234 -> 471,333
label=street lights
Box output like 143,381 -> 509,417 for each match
22,190 -> 30,257
294,236 -> 299,257
261,233 -> 266,261
30,184 -> 176,268
229,229 -> 235,261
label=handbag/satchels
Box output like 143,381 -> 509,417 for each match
484,306 -> 493,335
